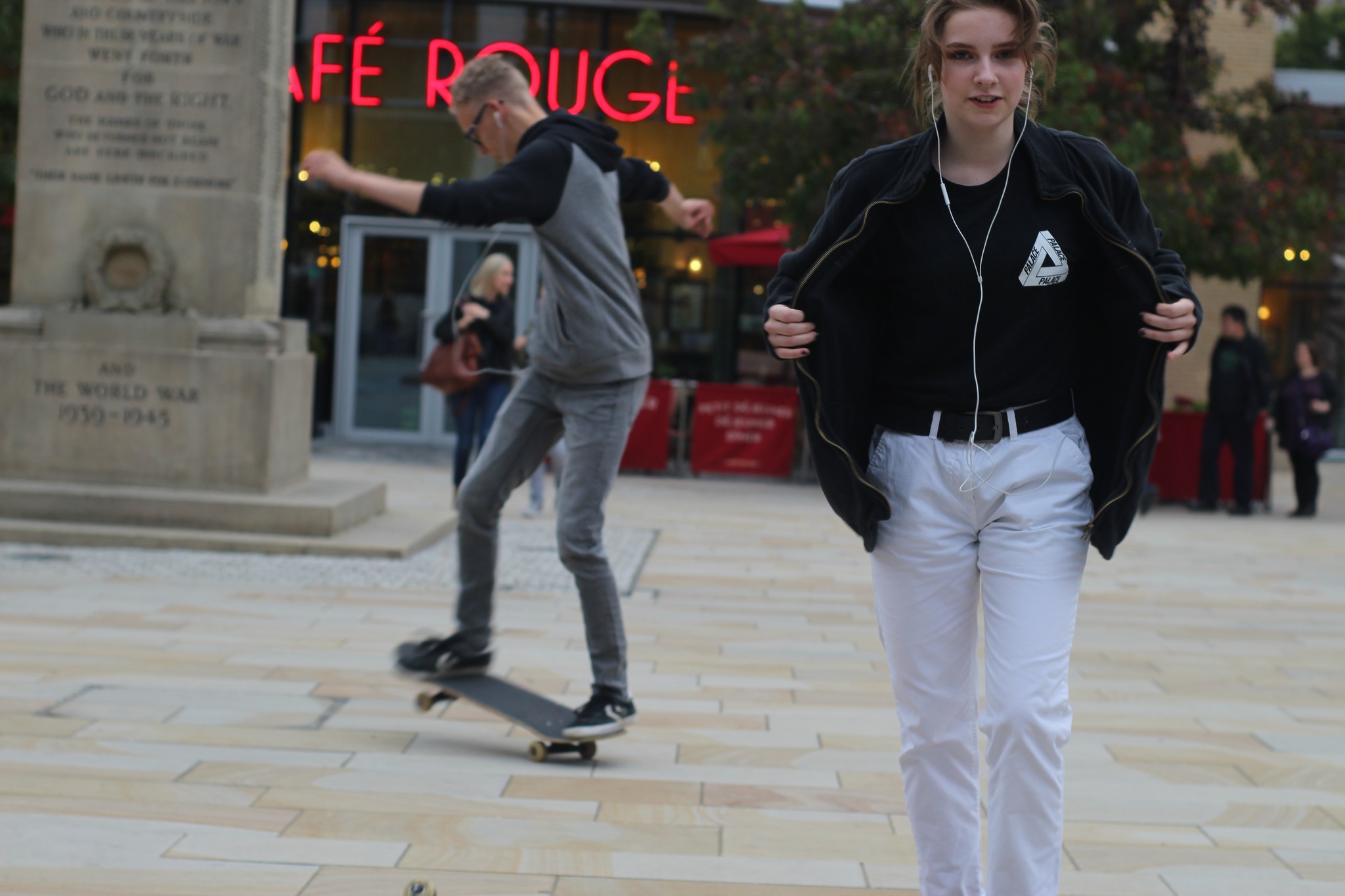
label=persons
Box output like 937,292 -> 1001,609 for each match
297,52 -> 717,747
1270,332 -> 1343,519
1192,301 -> 1273,515
520,433 -> 575,521
427,254 -> 532,512
764,0 -> 1201,896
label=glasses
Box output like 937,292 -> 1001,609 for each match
464,99 -> 504,142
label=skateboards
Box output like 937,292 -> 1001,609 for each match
392,638 -> 624,759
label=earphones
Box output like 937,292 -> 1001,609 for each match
1027,61 -> 1035,81
493,110 -> 503,129
928,63 -> 935,83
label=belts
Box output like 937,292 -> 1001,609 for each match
877,400 -> 1076,447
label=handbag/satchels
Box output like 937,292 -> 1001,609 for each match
1299,427 -> 1334,453
418,331 -> 481,393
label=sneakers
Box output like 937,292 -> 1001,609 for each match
394,638 -> 494,680
564,688 -> 636,739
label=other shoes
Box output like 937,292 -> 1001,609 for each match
1289,505 -> 1314,518
1186,500 -> 1216,511
1228,505 -> 1250,515
